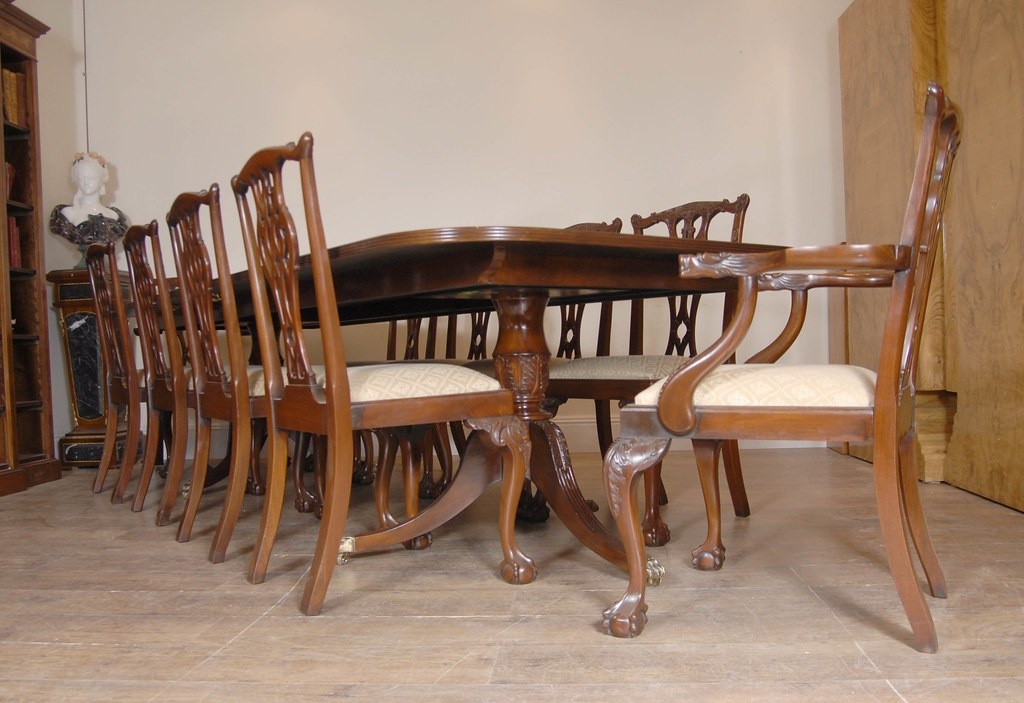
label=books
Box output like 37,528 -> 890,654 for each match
6,163 -> 16,200
8,217 -> 21,268
2,68 -> 27,127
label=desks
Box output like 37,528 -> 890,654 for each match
133,225 -> 795,587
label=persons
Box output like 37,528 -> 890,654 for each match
50,152 -> 129,226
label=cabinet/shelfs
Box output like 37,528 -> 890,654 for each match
0,0 -> 61,498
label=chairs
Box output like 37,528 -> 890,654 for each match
603,81 -> 962,653
86,182 -> 401,564
386,194 -> 766,522
230,131 -> 538,616
